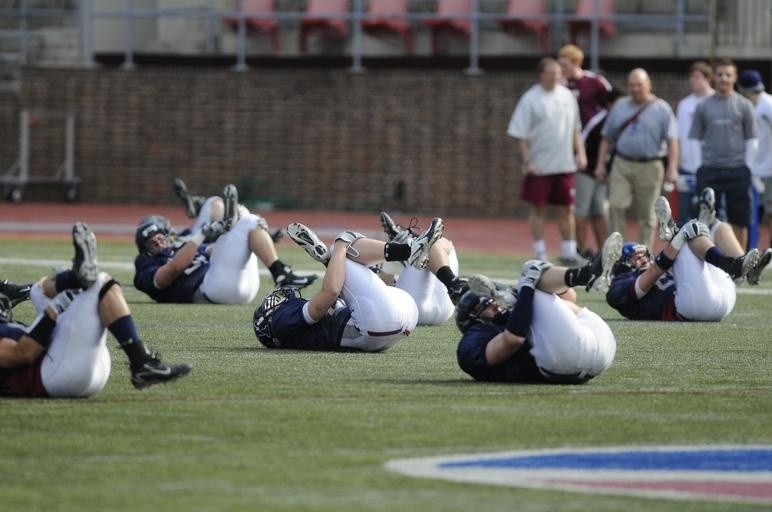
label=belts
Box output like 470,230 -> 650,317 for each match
541,366 -> 595,380
355,327 -> 410,336
617,153 -> 655,162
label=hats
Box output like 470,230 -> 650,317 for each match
738,69 -> 765,92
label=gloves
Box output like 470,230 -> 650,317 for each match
49,288 -> 77,313
682,218 -> 711,241
335,231 -> 365,257
521,259 -> 552,289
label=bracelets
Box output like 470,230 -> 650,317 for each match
523,157 -> 532,164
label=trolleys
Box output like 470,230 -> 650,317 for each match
0,110 -> 81,203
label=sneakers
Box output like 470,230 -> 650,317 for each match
559,232 -> 623,293
74,222 -> 99,287
468,274 -> 519,308
173,177 -> 443,288
748,248 -> 772,284
131,352 -> 191,389
730,250 -> 759,283
698,188 -> 716,227
655,196 -> 675,239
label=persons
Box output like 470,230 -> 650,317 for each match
365,211 -> 469,328
134,182 -> 317,306
594,68 -> 681,256
253,217 -> 446,353
690,59 -> 754,254
1,221 -> 191,399
732,71 -> 771,251
506,55 -> 589,267
573,88 -> 632,261
672,61 -> 725,236
457,232 -> 623,385
607,191 -> 772,323
554,44 -> 614,132
654,186 -> 771,287
139,179 -> 283,244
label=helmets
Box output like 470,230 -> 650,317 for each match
615,242 -> 652,271
456,290 -> 496,334
254,287 -> 302,348
136,213 -> 173,254
